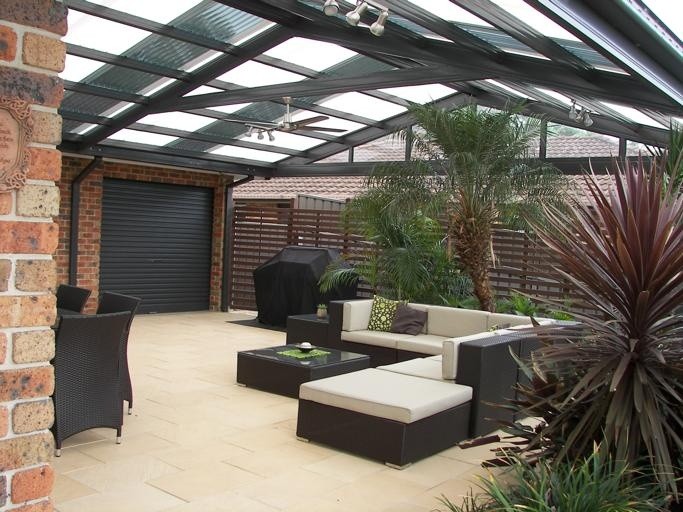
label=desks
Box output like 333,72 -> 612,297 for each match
286,313 -> 331,348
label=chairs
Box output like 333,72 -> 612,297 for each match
50,311 -> 131,458
57,284 -> 92,313
96,291 -> 141,416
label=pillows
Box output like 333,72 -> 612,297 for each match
391,301 -> 428,336
367,293 -> 409,331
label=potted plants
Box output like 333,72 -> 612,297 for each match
317,303 -> 328,319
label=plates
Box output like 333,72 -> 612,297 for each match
294,344 -> 317,353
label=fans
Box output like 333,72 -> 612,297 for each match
223,96 -> 349,134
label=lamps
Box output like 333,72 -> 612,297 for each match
243,123 -> 276,141
322,0 -> 390,36
568,100 -> 593,128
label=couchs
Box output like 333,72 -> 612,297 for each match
296,294 -> 582,470
